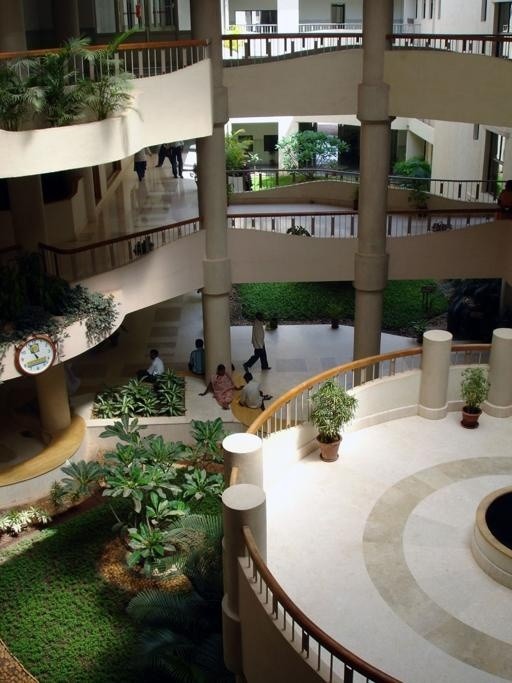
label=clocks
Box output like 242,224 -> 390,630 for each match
14,335 -> 56,375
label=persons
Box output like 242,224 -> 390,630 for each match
198,363 -> 242,409
155,144 -> 178,167
133,147 -> 152,181
187,338 -> 205,376
137,349 -> 165,383
238,372 -> 265,411
495,180 -> 512,221
166,141 -> 185,179
242,312 -> 271,373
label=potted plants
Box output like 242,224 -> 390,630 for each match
308,379 -> 357,462
460,367 -> 489,429
408,189 -> 430,217
413,320 -> 428,343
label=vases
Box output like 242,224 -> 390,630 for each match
332,319 -> 339,328
270,318 -> 277,328
